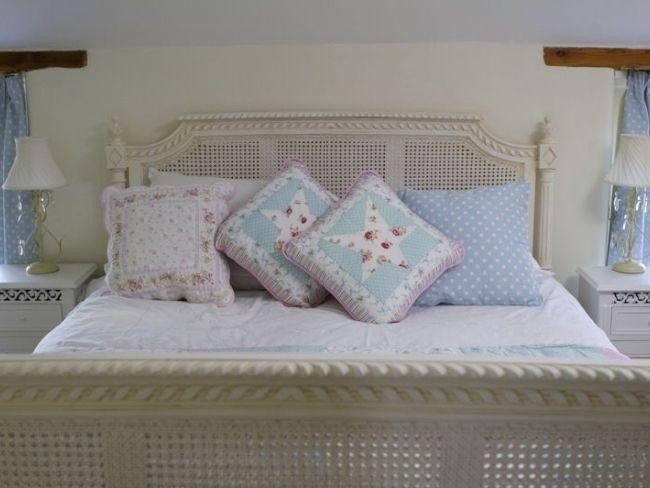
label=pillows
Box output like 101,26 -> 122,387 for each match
398,178 -> 545,308
101,182 -> 236,307
148,165 -> 273,291
282,170 -> 465,323
216,159 -> 339,307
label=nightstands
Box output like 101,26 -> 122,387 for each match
0,263 -> 97,354
575,265 -> 650,359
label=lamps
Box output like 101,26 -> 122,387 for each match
603,133 -> 650,273
1,136 -> 69,275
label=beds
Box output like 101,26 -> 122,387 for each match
0,110 -> 650,488
